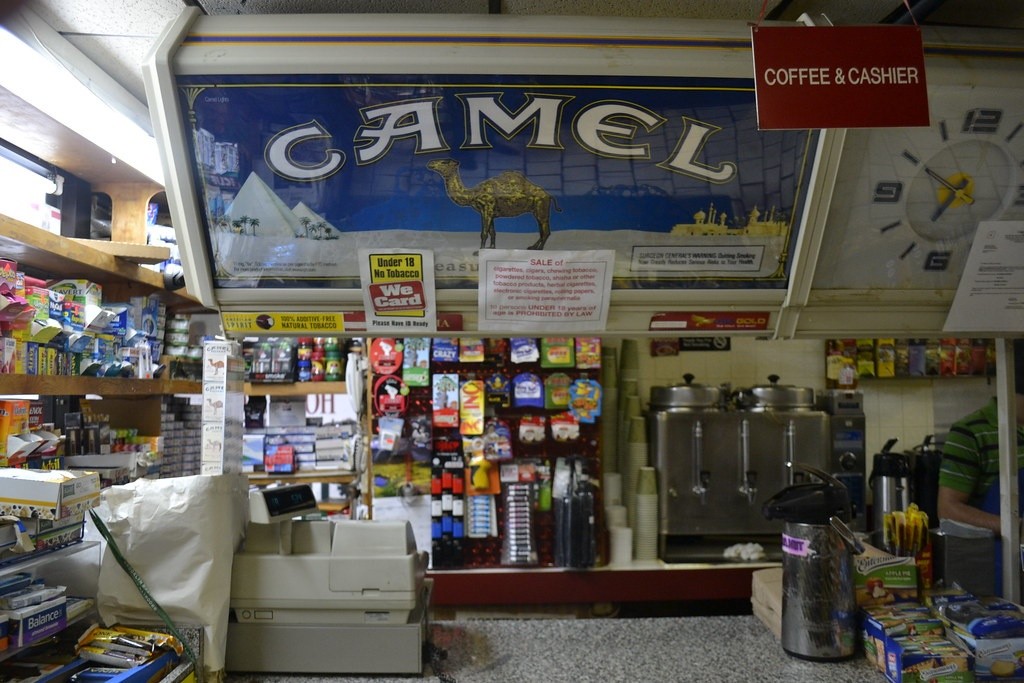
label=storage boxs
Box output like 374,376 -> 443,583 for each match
853,556 -> 1024,682
0,467 -> 101,563
0,256 -> 165,379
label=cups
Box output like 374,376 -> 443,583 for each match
602,338 -> 658,563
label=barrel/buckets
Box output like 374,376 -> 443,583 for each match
762,459 -> 866,661
903,434 -> 941,530
868,438 -> 917,551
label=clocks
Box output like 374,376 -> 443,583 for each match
809,81 -> 1024,289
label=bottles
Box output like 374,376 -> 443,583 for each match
296,337 -> 345,382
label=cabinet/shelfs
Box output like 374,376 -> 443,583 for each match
0,29 -> 218,395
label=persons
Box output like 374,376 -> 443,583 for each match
935,338 -> 1024,605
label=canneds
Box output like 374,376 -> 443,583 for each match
296,337 -> 343,382
